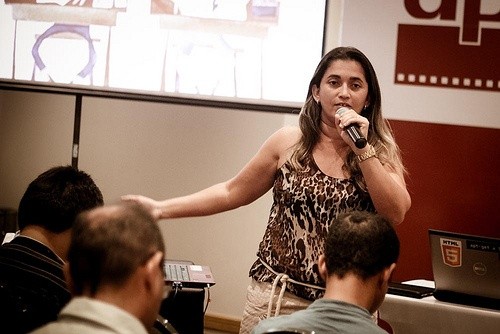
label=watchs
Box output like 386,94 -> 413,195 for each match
356,145 -> 378,163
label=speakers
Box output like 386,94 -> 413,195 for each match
147,287 -> 204,334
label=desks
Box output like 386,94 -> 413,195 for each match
376,279 -> 500,334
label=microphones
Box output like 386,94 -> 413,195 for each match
336,107 -> 367,149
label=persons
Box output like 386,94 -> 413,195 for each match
248,211 -> 401,334
0,165 -> 105,334
121,46 -> 412,334
29,198 -> 167,334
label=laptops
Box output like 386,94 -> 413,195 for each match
428,229 -> 500,309
163,264 -> 216,287
